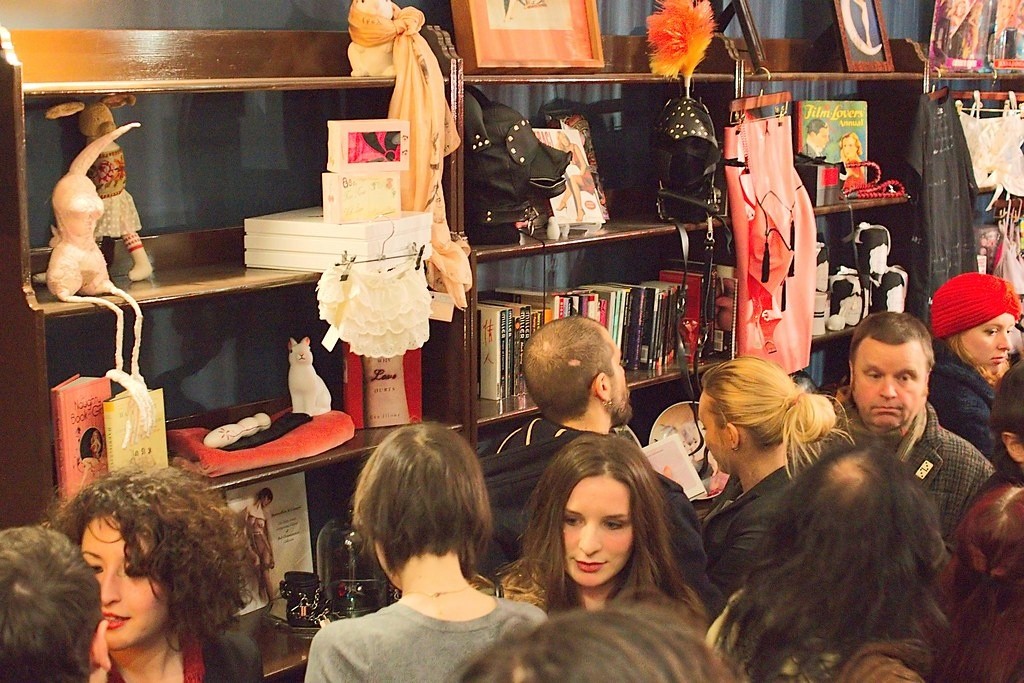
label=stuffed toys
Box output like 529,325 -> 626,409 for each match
346,0 -> 396,76
287,337 -> 331,417
44,93 -> 153,282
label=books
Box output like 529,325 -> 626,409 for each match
477,259 -> 738,402
640,434 -> 708,501
51,373 -> 112,504
103,387 -> 169,472
796,99 -> 870,187
534,127 -> 606,230
929,0 -> 1024,76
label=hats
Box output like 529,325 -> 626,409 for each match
930,272 -> 1021,340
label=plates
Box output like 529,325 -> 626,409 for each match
647,401 -> 731,500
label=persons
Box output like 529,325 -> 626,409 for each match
302,421 -> 549,683
244,486 -> 276,604
555,130 -> 596,223
448,432 -> 754,683
0,465 -> 266,683
697,357 -> 856,624
474,316 -> 708,600
705,272 -> 1023,683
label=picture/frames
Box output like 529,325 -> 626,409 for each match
452,0 -> 604,76
829,0 -> 896,73
730,0 -> 771,74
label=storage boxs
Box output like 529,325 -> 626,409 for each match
244,119 -> 436,272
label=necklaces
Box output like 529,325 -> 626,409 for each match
404,585 -> 469,598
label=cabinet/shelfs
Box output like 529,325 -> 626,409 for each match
433,24 -> 741,547
712,32 -> 931,404
1,27 -> 472,683
905,38 -> 1024,300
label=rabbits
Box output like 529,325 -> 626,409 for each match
287,337 -> 332,416
347,0 -> 394,78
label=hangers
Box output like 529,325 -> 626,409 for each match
334,216 -> 428,282
992,195 -> 1024,221
729,66 -> 792,125
925,67 -> 1023,120
